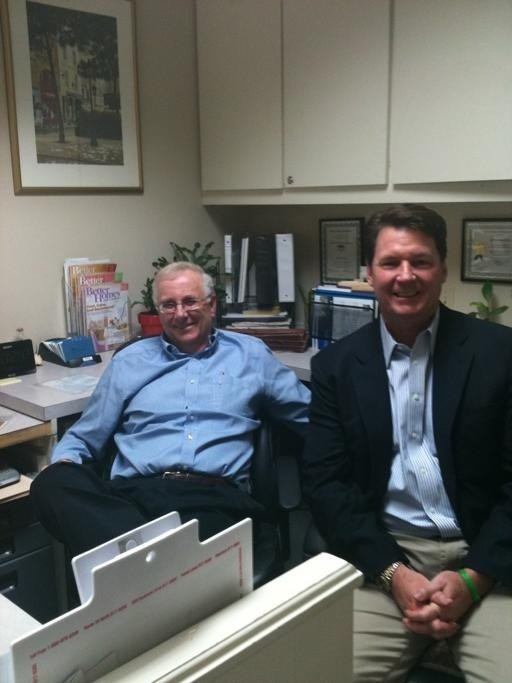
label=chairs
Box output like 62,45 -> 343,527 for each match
255,416 -> 303,584
304,519 -> 464,680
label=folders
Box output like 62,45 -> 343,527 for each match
274,232 -> 295,303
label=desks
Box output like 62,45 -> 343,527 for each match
0,407 -> 69,617
0,594 -> 42,683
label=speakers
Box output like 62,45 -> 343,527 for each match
0,339 -> 36,379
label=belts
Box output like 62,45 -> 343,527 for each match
152,470 -> 206,480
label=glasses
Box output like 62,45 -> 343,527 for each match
159,294 -> 211,314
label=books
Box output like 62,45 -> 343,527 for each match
222,233 -> 295,329
64,257 -> 131,352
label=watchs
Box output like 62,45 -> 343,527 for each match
376,560 -> 403,594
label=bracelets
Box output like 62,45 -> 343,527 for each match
300,204 -> 512,683
459,569 -> 480,605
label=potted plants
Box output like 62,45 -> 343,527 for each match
129,277 -> 163,337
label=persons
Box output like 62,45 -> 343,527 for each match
29,261 -> 313,591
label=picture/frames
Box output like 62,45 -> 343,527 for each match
318,217 -> 363,286
0,0 -> 144,196
461,215 -> 512,284
